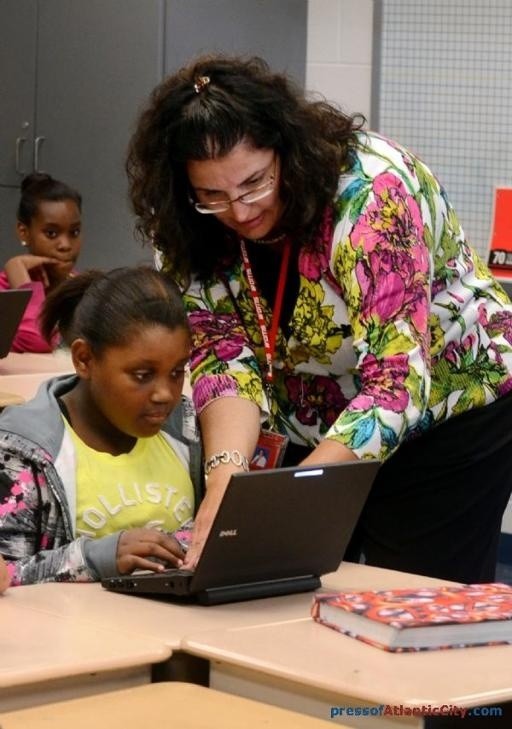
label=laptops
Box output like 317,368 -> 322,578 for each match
1,288 -> 33,360
100,459 -> 380,605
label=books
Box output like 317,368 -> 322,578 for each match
311,582 -> 512,653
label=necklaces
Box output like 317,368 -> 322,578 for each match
65,272 -> 75,280
249,234 -> 286,245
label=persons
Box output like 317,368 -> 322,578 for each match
0,172 -> 85,354
0,268 -> 205,588
0,556 -> 9,594
251,448 -> 266,467
125,50 -> 511,585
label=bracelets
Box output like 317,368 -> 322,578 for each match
201,448 -> 250,481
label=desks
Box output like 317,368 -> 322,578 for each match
1,347 -> 83,401
1,391 -> 25,413
181,577 -> 511,729
1,676 -> 342,729
1,579 -> 170,710
56,554 -> 467,685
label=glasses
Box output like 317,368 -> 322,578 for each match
186,145 -> 278,217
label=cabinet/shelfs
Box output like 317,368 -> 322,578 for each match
0,0 -> 312,285
